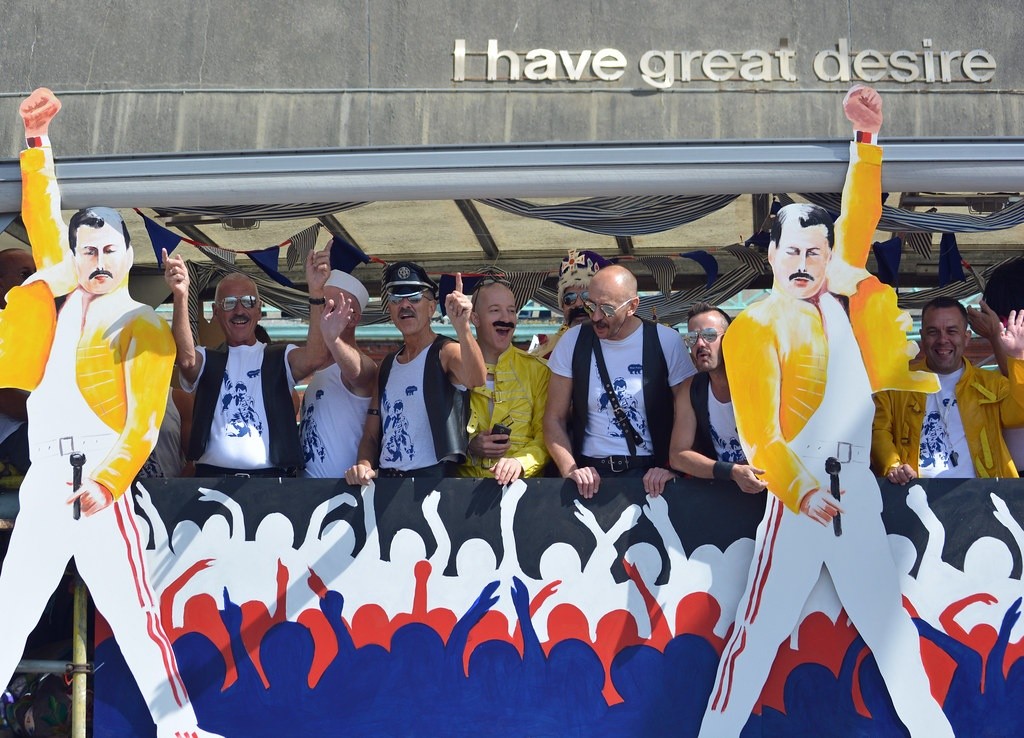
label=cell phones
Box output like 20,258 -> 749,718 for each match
491,423 -> 512,444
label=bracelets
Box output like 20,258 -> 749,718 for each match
669,467 -> 686,476
309,296 -> 326,305
713,461 -> 734,481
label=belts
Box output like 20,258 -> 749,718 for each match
577,455 -> 655,473
194,463 -> 295,477
378,467 -> 434,478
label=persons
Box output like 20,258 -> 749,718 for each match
668,301 -> 769,494
543,265 -> 698,499
255,324 -> 300,413
136,387 -> 195,477
873,297 -> 1024,485
455,279 -> 552,485
298,269 -> 379,478
162,239 -> 333,477
532,248 -> 612,359
0,249 -> 37,474
344,261 -> 486,485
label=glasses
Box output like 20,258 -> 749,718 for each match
682,328 -> 724,347
215,296 -> 260,312
388,292 -> 431,305
562,291 -> 590,306
584,299 -> 634,318
471,280 -> 512,312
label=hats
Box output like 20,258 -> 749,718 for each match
557,248 -> 611,311
323,268 -> 369,315
385,261 -> 438,298
